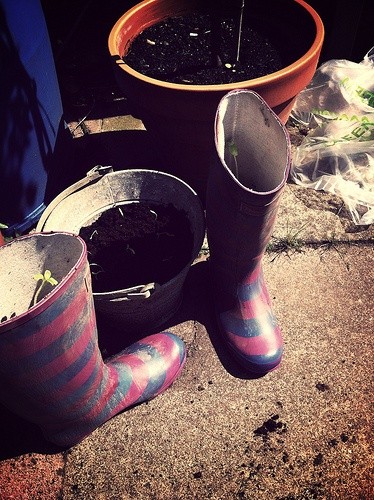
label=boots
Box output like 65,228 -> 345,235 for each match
206,88 -> 293,370
0,233 -> 189,450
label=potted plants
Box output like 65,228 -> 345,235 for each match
109,0 -> 325,182
35,164 -> 205,332
